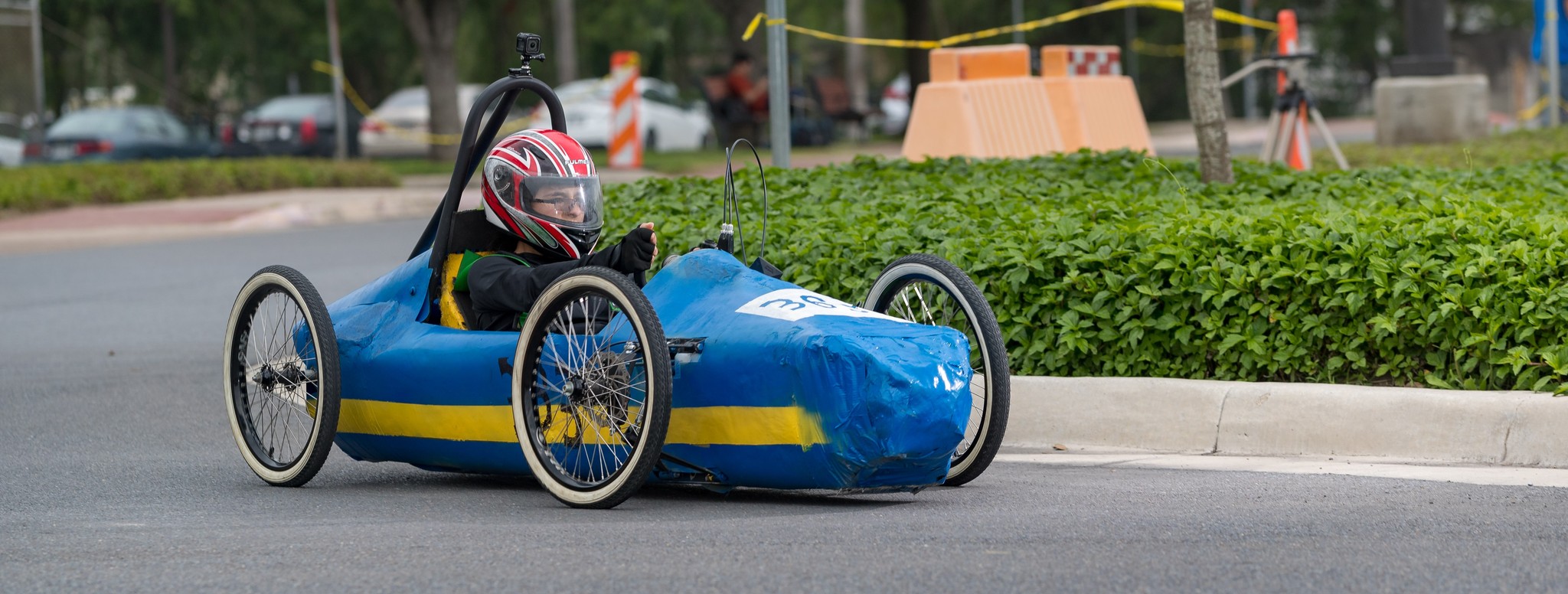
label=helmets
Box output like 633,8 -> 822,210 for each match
481,129 -> 604,259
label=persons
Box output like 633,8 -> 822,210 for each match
686,55 -> 735,147
454,128 -> 715,335
730,47 -> 769,146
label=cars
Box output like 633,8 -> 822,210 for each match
218,94 -> 367,158
353,81 -> 527,164
18,103 -> 231,164
525,72 -> 718,157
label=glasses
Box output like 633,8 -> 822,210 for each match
528,198 -> 590,212
